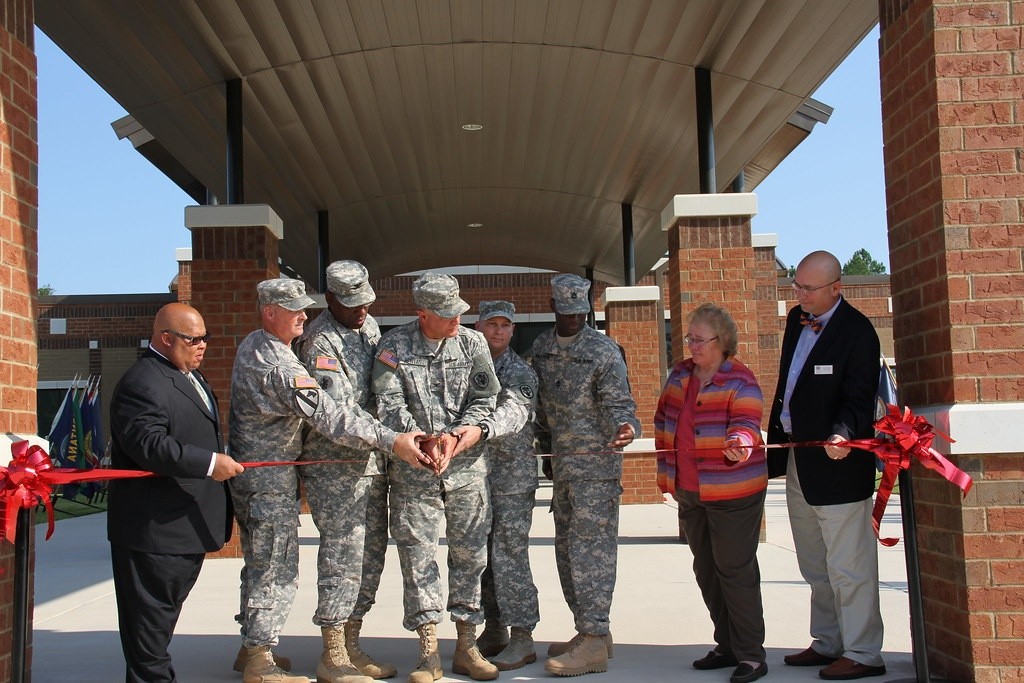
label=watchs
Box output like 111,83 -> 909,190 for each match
476,422 -> 489,441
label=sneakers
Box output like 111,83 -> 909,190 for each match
784,647 -> 843,666
819,656 -> 886,679
548,630 -> 614,658
544,635 -> 608,676
494,627 -> 537,671
476,620 -> 509,657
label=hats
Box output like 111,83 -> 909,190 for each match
479,301 -> 516,322
551,273 -> 591,315
326,260 -> 376,308
257,278 -> 317,311
412,272 -> 471,320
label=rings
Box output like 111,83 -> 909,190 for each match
834,455 -> 838,460
732,457 -> 736,459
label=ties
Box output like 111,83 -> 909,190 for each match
186,373 -> 215,418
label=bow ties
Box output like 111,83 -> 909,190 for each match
800,314 -> 823,335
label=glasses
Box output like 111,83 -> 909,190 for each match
684,333 -> 718,345
791,277 -> 840,295
160,329 -> 211,345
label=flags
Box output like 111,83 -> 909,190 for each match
46,382 -> 112,501
874,360 -> 898,473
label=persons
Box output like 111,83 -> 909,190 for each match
372,272 -> 502,683
653,302 -> 768,683
293,260 -> 428,683
450,300 -> 539,671
227,278 -> 430,683
106,302 -> 244,683
766,250 -> 887,679
531,273 -> 642,676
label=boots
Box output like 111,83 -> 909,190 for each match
243,645 -> 312,683
452,621 -> 499,681
317,625 -> 374,683
408,624 -> 443,683
344,616 -> 397,679
232,643 -> 291,672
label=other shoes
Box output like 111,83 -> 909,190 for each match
693,650 -> 738,670
730,661 -> 768,683
